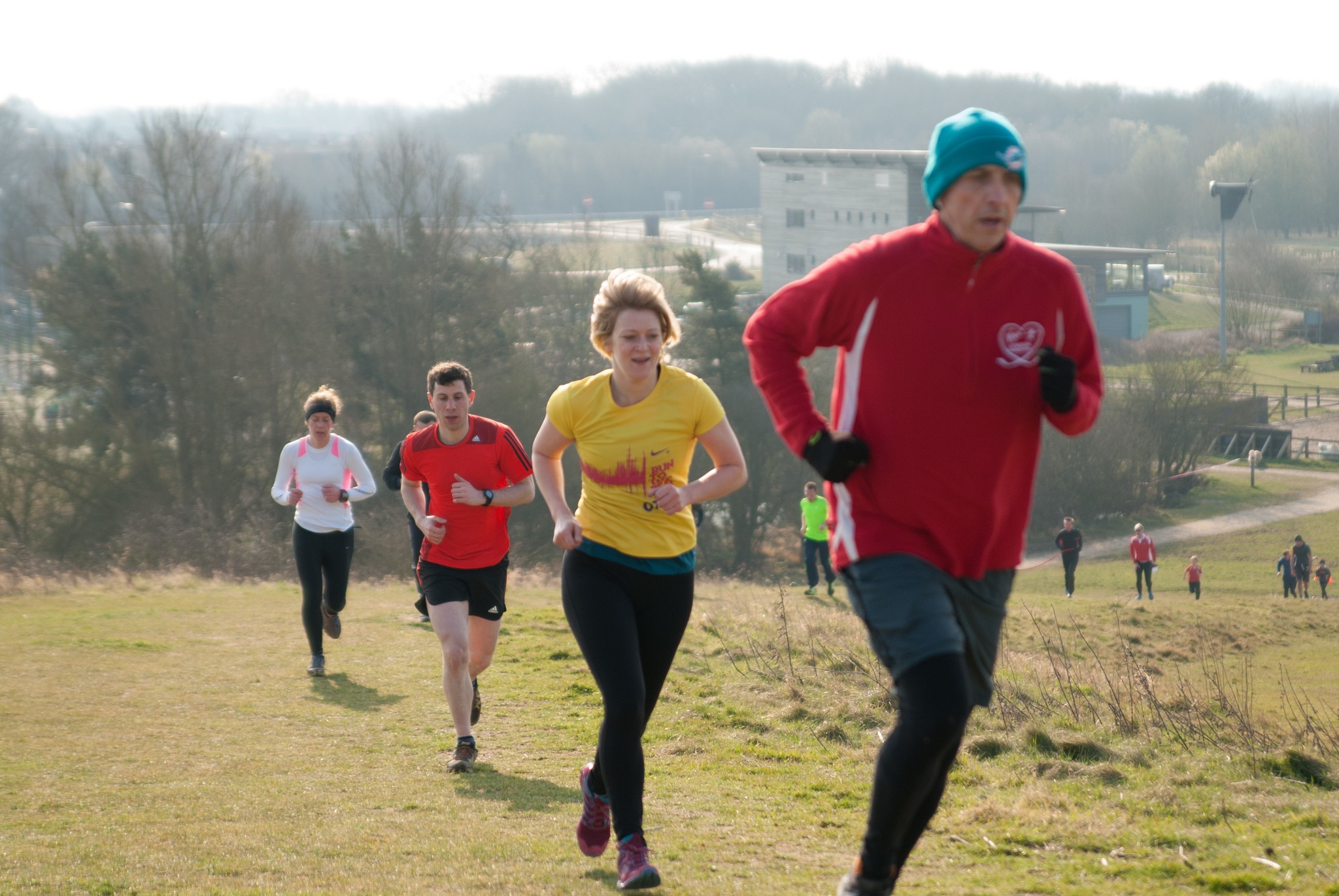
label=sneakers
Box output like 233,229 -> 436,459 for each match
804,586 -> 817,595
447,740 -> 478,771
616,833 -> 661,890
307,654 -> 325,676
576,762 -> 611,857
470,678 -> 481,726
321,594 -> 341,639
827,580 -> 835,595
1060,581 -> 1336,605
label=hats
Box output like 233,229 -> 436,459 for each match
1294,534 -> 1302,541
1319,559 -> 1326,564
921,107 -> 1029,210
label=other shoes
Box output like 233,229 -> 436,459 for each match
418,593 -> 430,622
834,871 -> 892,896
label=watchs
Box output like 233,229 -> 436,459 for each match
482,489 -> 493,507
339,489 -> 349,502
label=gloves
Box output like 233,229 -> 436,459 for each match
803,428 -> 871,484
1038,340 -> 1077,413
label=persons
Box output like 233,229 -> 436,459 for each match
742,106 -> 1106,895
1182,555 -> 1202,603
800,482 -> 835,597
398,362 -> 535,773
1314,559 -> 1331,600
1130,522 -> 1157,599
1055,517 -> 1083,598
1277,549 -> 1319,598
383,411 -> 465,622
270,385 -> 376,677
531,267 -> 748,890
1289,534 -> 1313,600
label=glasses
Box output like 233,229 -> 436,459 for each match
1135,528 -> 1142,532
